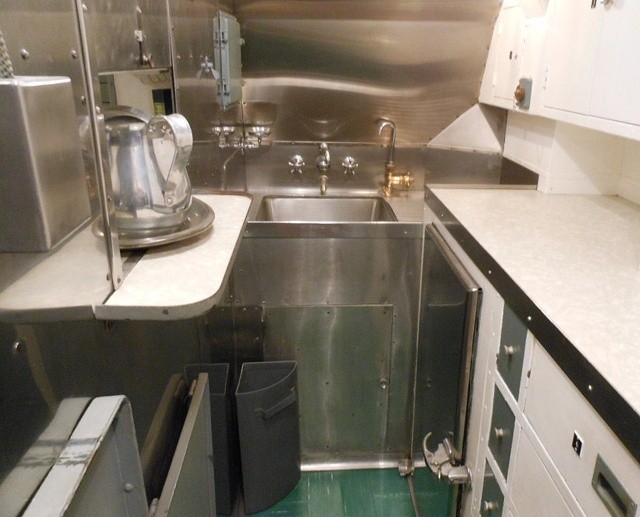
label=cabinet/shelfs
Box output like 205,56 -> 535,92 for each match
479,0 -> 546,116
471,313 -> 640,517
544,0 -> 639,139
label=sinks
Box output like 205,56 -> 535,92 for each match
257,196 -> 398,222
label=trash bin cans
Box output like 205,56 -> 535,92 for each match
234,360 -> 300,515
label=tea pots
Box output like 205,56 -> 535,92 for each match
81,106 -> 192,230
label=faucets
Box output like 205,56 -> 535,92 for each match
315,141 -> 333,173
377,120 -> 414,196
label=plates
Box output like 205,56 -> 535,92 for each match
87,195 -> 218,248
